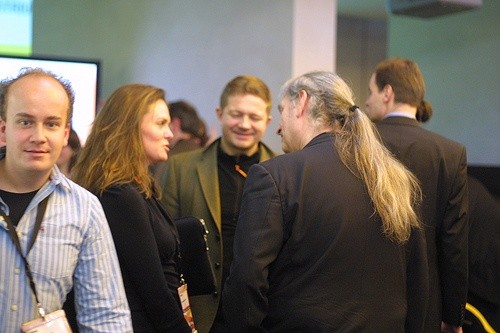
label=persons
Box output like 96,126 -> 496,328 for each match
464,171 -> 500,333
57,128 -> 83,177
165,102 -> 217,157
66,84 -> 195,333
362,57 -> 473,333
413,99 -> 431,124
160,74 -> 280,333
0,68 -> 136,333
207,69 -> 433,333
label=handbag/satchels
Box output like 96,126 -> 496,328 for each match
173,215 -> 218,297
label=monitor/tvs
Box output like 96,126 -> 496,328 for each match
0,55 -> 101,148
